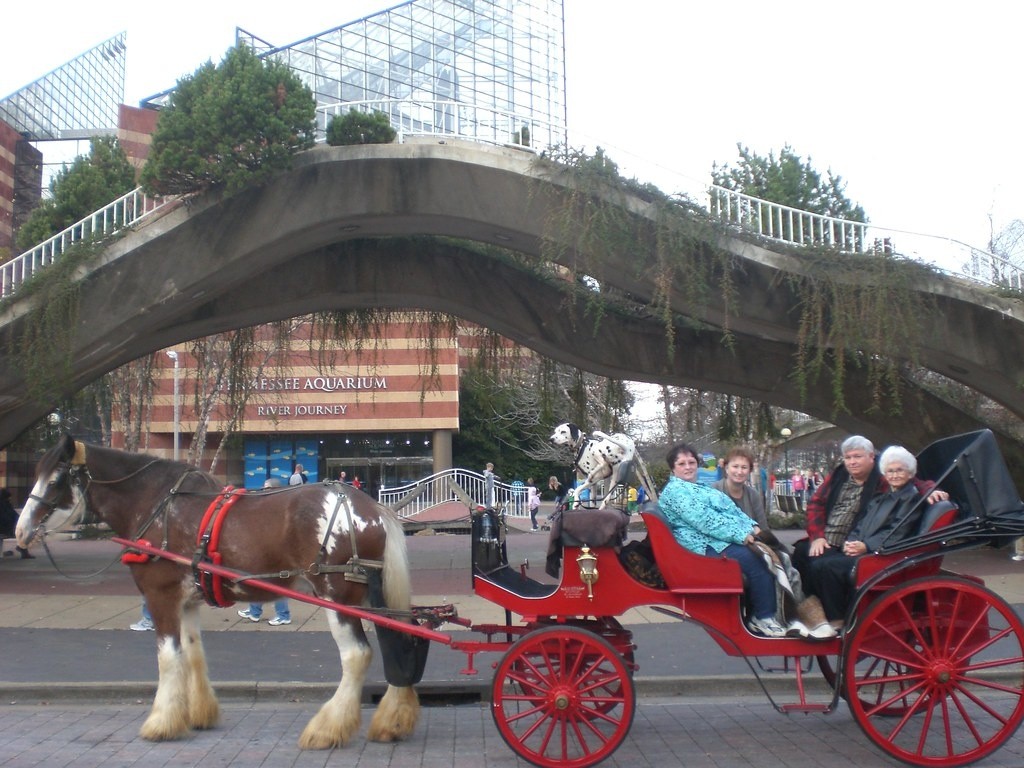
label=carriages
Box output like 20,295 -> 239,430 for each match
12,429 -> 1024,767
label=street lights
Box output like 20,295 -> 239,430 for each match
780,427 -> 791,517
167,350 -> 180,463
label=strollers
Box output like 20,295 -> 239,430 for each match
539,493 -> 574,531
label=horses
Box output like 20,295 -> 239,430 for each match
14,432 -> 420,751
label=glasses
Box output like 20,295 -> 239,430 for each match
885,469 -> 909,475
675,460 -> 696,466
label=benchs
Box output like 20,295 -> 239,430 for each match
639,502 -> 744,593
545,480 -> 632,579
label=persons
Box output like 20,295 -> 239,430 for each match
714,458 -> 724,481
756,467 -> 775,512
528,487 -> 540,530
288,464 -> 307,486
129,598 -> 155,631
711,447 -> 790,557
339,471 -> 346,482
483,463 -> 501,508
537,476 -> 568,509
628,483 -> 644,515
352,476 -> 361,490
237,478 -> 291,625
792,468 -> 815,512
657,443 -> 788,637
0,487 -> 36,559
791,435 -> 949,632
510,478 -> 542,514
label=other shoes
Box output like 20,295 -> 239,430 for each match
786,621 -> 808,638
808,622 -> 841,640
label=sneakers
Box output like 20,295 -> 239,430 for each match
268,617 -> 291,626
742,612 -> 785,637
130,617 -> 155,631
237,610 -> 259,622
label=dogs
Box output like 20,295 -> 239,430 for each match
620,538 -> 665,590
550,421 -> 635,510
